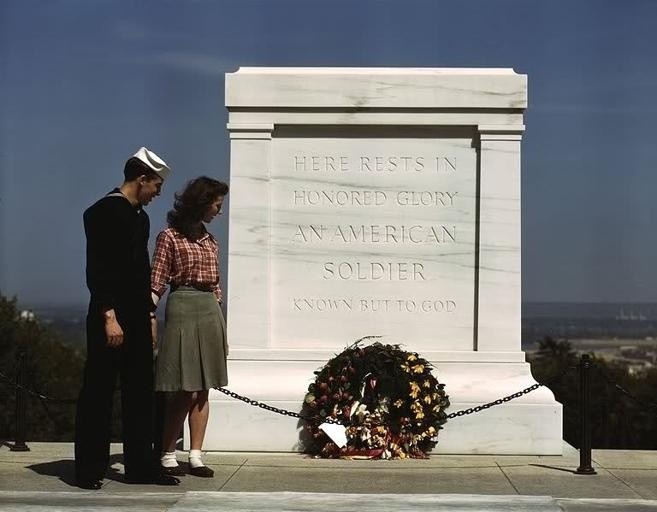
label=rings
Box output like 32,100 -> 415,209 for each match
152,342 -> 154,345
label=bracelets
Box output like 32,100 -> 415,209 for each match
149,313 -> 156,320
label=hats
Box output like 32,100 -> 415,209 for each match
132,146 -> 171,180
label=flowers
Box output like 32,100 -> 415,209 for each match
296,341 -> 453,461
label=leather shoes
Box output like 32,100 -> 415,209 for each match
157,476 -> 180,485
88,480 -> 101,489
161,466 -> 185,476
191,467 -> 214,477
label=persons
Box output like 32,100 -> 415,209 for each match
73,146 -> 181,489
148,174 -> 229,478
